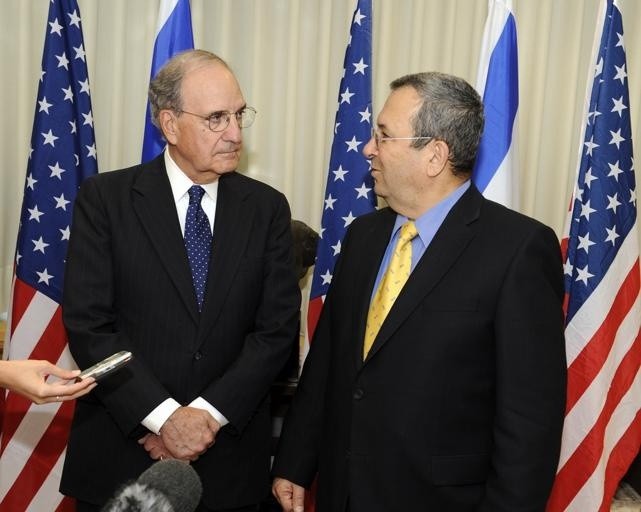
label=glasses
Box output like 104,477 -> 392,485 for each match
371,127 -> 431,147
180,106 -> 257,132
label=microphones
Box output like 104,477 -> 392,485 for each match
111,459 -> 204,511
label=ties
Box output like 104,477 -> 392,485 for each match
363,222 -> 418,363
184,185 -> 213,312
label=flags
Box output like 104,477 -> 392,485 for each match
543,1 -> 641,509
469,0 -> 521,213
139,0 -> 195,167
1,1 -> 100,511
302,0 -> 378,511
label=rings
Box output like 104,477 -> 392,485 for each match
56,393 -> 61,402
160,456 -> 163,461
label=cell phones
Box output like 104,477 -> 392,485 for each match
77,349 -> 134,382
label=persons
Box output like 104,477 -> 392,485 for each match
56,45 -> 302,511
1,358 -> 97,406
270,70 -> 570,511
